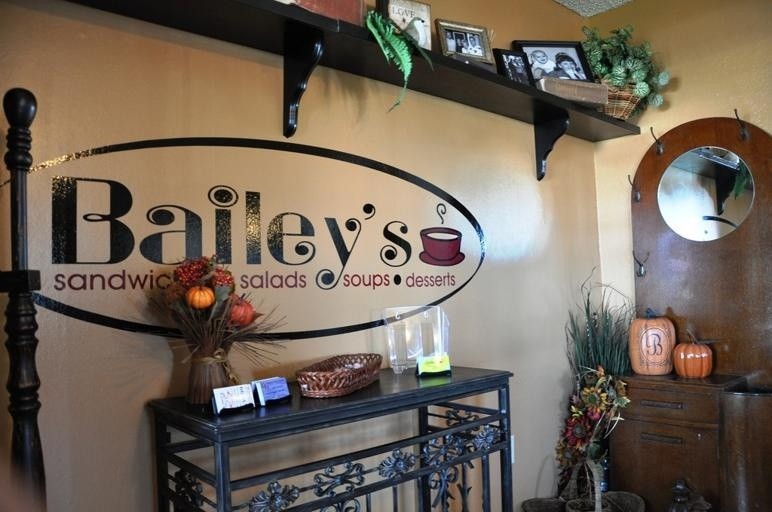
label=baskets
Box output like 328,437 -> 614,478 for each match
523,459 -> 646,512
597,78 -> 643,118
295,352 -> 382,398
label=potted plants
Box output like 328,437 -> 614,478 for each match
581,25 -> 670,121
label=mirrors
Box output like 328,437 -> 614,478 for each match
654,147 -> 757,245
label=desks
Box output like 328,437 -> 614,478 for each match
144,365 -> 514,512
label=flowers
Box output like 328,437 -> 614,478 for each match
554,269 -> 641,497
129,256 -> 288,405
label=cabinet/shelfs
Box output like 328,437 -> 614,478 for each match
608,376 -> 747,511
72,0 -> 640,181
672,149 -> 740,216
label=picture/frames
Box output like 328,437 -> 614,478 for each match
377,1 -> 431,49
495,47 -> 533,86
435,18 -> 494,63
512,39 -> 595,84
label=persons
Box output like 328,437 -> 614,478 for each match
446,30 -> 483,56
534,52 -> 585,81
507,55 -> 525,83
530,50 -> 557,79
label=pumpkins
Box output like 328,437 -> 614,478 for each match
673,340 -> 712,381
227,296 -> 254,328
185,285 -> 213,308
